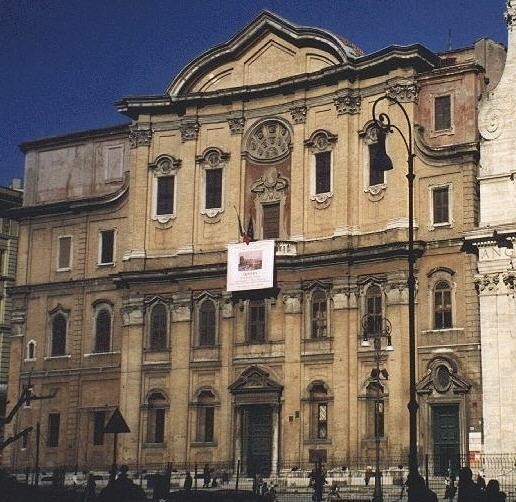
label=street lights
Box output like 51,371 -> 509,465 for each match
371,94 -> 423,502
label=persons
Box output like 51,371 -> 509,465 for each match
442,467 -> 456,499
202,463 -> 211,488
182,471 -> 193,490
86,472 -> 96,494
321,465 -> 327,483
